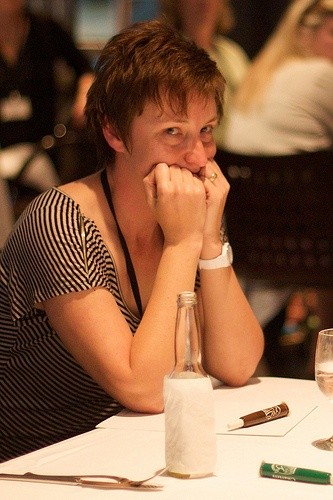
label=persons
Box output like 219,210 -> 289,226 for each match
0,0 -> 332,463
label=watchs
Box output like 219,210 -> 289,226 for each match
198,241 -> 234,271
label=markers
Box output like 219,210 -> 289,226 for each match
226,402 -> 289,431
258,460 -> 332,486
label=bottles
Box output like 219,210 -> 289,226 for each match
165,291 -> 214,479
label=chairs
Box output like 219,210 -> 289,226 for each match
212,145 -> 333,289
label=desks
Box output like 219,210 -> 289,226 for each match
0,377 -> 333,500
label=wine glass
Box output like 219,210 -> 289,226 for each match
314,327 -> 333,452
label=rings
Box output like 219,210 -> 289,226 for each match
208,173 -> 217,182
